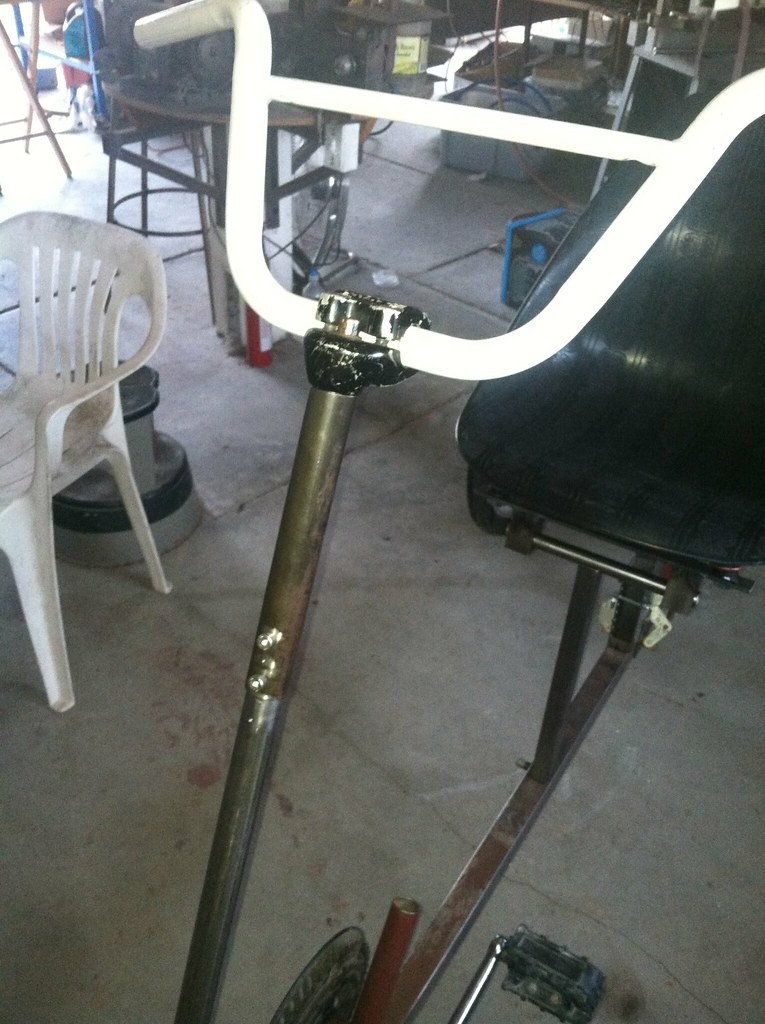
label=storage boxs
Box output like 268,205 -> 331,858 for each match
438,83 -> 564,180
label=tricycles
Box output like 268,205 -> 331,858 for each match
124,0 -> 765,1024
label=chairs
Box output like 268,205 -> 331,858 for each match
0,211 -> 175,712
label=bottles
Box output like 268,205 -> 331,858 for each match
302,269 -> 325,300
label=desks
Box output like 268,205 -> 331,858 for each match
101,80 -> 378,356
